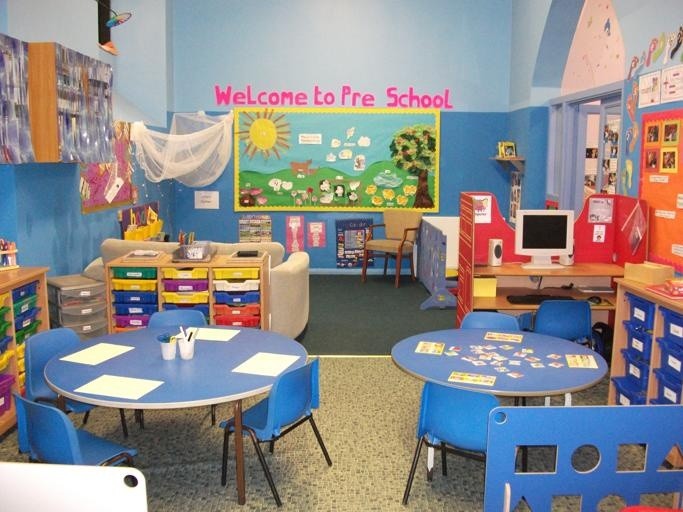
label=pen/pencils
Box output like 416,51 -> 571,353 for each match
0,239 -> 16,266
179,325 -> 199,342
179,228 -> 194,259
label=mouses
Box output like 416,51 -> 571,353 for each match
587,296 -> 601,304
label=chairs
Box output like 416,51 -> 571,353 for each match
533,300 -> 592,350
460,312 -> 520,331
401,380 -> 527,506
135,310 -> 216,426
362,208 -> 422,288
219,358 -> 333,507
12,392 -> 139,486
25,328 -> 129,439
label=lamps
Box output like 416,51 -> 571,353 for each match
95,0 -> 133,28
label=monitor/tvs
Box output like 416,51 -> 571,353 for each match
515,210 -> 574,269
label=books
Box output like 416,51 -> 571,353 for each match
125,250 -> 164,263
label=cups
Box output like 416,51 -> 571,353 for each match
159,342 -> 176,360
178,338 -> 194,360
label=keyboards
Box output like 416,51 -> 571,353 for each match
506,294 -> 576,304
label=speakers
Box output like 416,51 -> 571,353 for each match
559,239 -> 575,266
488,239 -> 503,267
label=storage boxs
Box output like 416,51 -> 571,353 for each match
20,386 -> 26,396
0,306 -> 11,325
112,303 -> 158,315
624,292 -> 655,330
161,279 -> 208,292
623,320 -> 651,365
0,321 -> 12,340
160,268 -> 208,278
178,241 -> 211,260
213,303 -> 261,315
213,291 -> 260,303
658,306 -> 683,347
162,303 -> 209,317
18,358 -> 25,372
213,268 -> 260,279
112,278 -> 158,290
213,280 -> 261,291
655,337 -> 683,385
16,343 -> 25,359
610,377 -> 646,405
15,307 -> 41,330
161,291 -> 209,303
111,267 -> 157,278
0,350 -> 15,372
649,399 -> 658,404
473,275 -> 496,297
0,293 -> 10,309
19,371 -> 25,387
516,313 -> 532,330
621,349 -> 649,390
16,320 -> 42,344
47,278 -> 108,341
14,293 -> 38,319
653,368 -> 682,404
0,374 -> 16,416
114,326 -> 143,332
111,290 -> 158,304
112,314 -> 150,327
0,336 -> 13,355
12,283 -> 39,303
213,315 -> 260,327
205,317 -> 209,323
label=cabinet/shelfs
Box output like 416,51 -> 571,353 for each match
609,278 -> 683,470
106,257 -> 268,335
0,267 -> 49,436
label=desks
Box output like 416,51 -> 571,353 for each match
473,264 -> 624,309
391,328 -> 608,483
43,325 -> 308,505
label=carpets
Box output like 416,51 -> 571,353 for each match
294,275 -> 457,356
1,356 -> 673,511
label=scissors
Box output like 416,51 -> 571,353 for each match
157,332 -> 170,342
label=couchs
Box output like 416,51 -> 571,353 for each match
83,239 -> 309,339
